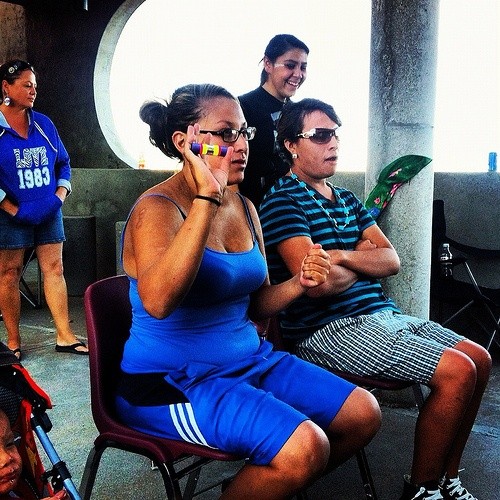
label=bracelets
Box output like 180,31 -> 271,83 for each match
195,195 -> 222,207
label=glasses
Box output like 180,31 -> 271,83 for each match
296,126 -> 342,143
199,126 -> 256,143
14,60 -> 35,74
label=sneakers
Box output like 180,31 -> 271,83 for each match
402,471 -> 478,500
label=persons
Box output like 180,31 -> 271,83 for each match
112,84 -> 382,500
236,34 -> 309,210
0,59 -> 89,361
257,98 -> 492,500
0,410 -> 67,500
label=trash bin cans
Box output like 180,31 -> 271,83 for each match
62,216 -> 96,296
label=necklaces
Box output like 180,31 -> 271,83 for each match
289,167 -> 350,231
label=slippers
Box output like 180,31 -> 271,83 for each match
55,338 -> 89,355
8,347 -> 22,361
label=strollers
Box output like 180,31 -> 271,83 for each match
0,342 -> 82,500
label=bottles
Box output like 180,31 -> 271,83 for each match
440,243 -> 453,282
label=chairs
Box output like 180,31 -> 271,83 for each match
430,200 -> 500,350
270,315 -> 424,500
78,274 -> 250,500
